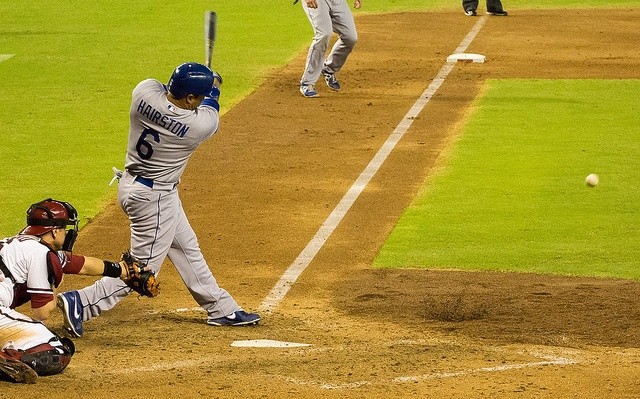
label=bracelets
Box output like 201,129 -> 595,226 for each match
101,258 -> 123,279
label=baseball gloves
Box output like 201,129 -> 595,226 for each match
119,250 -> 160,298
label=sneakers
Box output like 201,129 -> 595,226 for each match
208,309 -> 261,326
487,8 -> 508,15
56,290 -> 84,338
464,9 -> 476,16
319,68 -> 338,89
299,84 -> 320,97
0,352 -> 39,384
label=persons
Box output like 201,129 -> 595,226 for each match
0,199 -> 145,383
294,0 -> 363,99
461,0 -> 508,15
56,61 -> 262,338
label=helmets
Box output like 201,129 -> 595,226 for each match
18,198 -> 80,252
166,62 -> 214,100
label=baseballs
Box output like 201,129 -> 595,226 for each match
585,173 -> 599,186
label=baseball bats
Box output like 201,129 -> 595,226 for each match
205,12 -> 217,69
